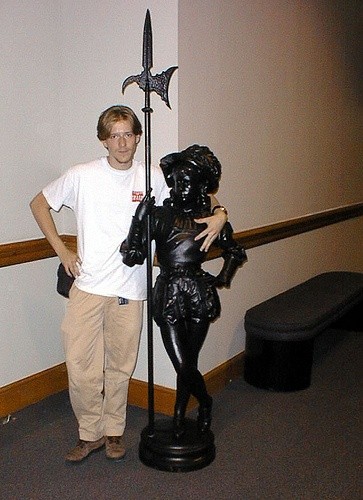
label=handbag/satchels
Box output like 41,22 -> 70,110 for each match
56,263 -> 75,299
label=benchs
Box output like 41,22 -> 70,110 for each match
244,271 -> 363,390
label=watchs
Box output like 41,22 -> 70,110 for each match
211,204 -> 228,217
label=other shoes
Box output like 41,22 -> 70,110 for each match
104,435 -> 126,459
64,436 -> 107,461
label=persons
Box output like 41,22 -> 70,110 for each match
30,105 -> 228,463
120,144 -> 249,440
